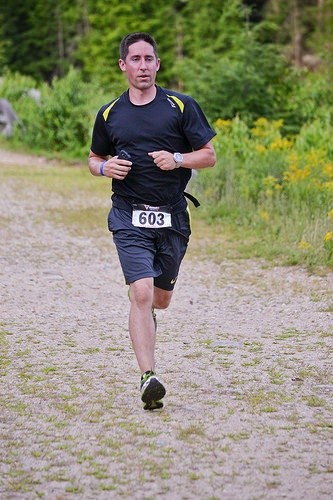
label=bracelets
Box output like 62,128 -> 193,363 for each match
99,161 -> 107,176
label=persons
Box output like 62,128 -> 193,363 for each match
87,29 -> 219,414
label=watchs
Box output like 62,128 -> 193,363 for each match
173,152 -> 183,168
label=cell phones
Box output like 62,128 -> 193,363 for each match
117,149 -> 131,161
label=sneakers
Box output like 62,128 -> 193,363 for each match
127,286 -> 157,332
140,367 -> 166,410
